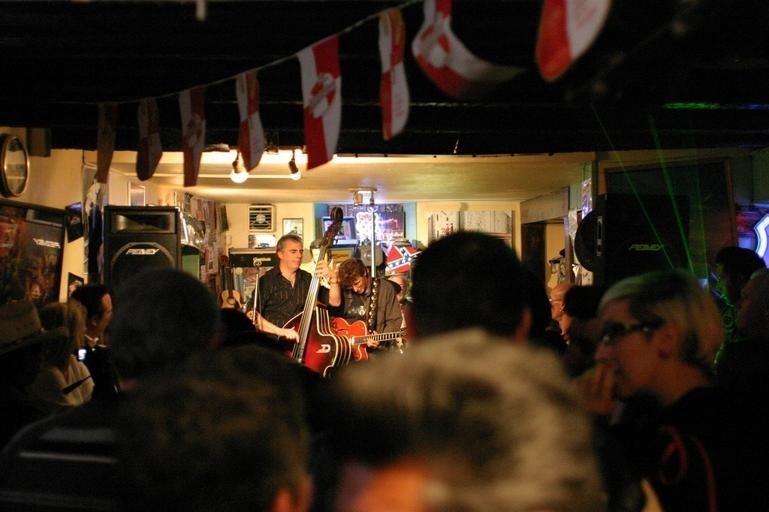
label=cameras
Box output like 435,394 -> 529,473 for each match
76,344 -> 117,393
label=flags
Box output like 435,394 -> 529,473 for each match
534,0 -> 611,83
377,7 -> 410,141
177,84 -> 207,188
93,102 -> 116,184
411,0 -> 527,100
135,95 -> 163,181
235,68 -> 268,172
295,35 -> 343,171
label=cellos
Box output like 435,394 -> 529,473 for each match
278,207 -> 351,378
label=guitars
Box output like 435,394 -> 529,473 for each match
331,317 -> 408,363
221,255 -> 243,308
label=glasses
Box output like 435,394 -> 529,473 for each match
602,320 -> 663,345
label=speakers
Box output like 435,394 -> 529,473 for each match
103,204 -> 182,287
593,191 -> 690,290
0,199 -> 69,302
